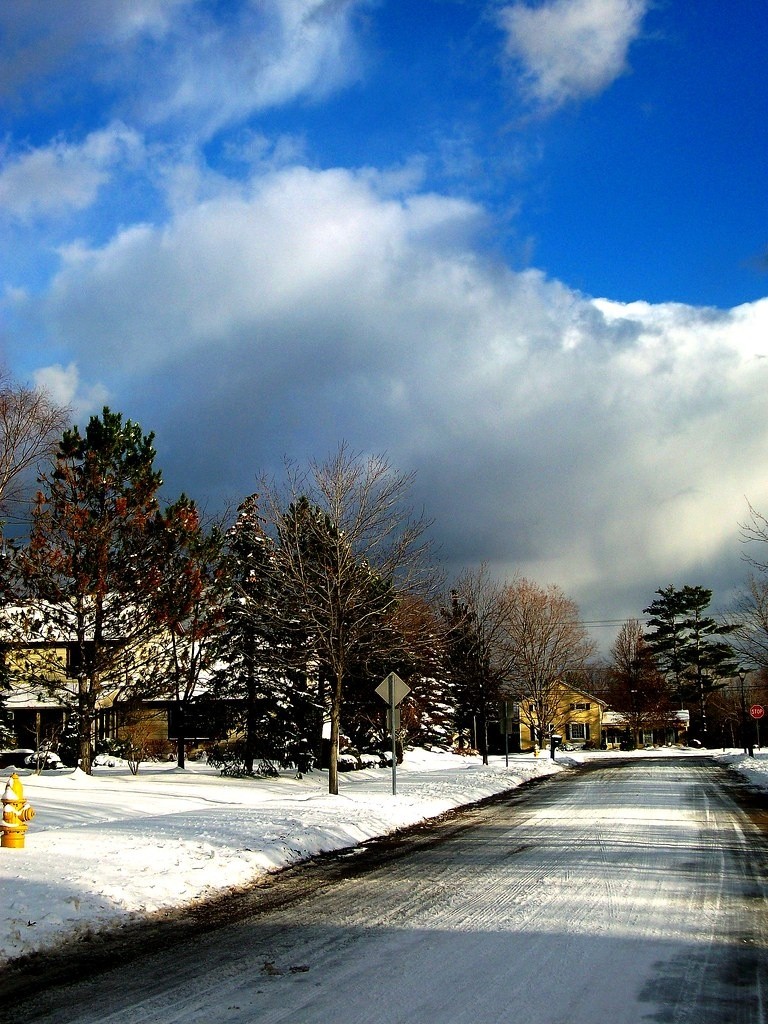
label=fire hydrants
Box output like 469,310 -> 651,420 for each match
0,772 -> 36,849
534,744 -> 541,757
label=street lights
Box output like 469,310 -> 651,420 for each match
737,667 -> 749,754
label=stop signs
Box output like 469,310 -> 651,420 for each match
750,704 -> 764,719
